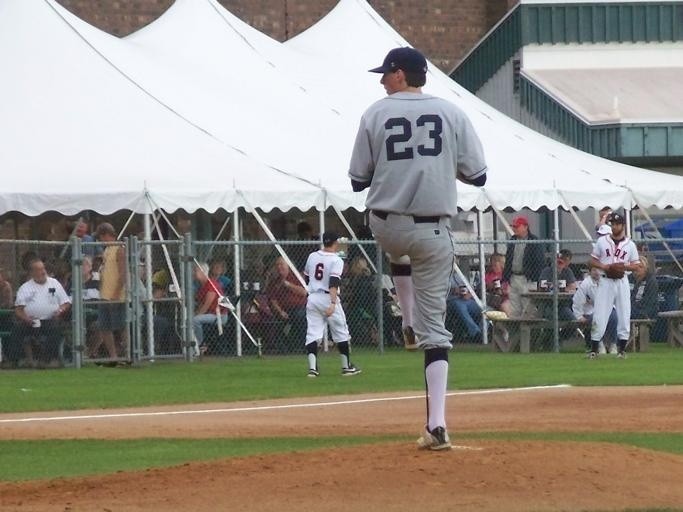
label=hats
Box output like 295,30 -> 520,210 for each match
367,46 -> 428,73
510,217 -> 529,227
93,223 -> 118,236
608,213 -> 626,224
323,231 -> 341,242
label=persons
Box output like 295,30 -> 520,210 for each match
345,47 -> 488,454
2,207 -> 657,370
301,230 -> 362,379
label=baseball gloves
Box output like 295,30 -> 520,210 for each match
607,262 -> 625,279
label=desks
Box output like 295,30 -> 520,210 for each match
506,292 -> 575,352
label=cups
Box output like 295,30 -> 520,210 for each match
460,286 -> 467,295
540,280 -> 549,291
494,279 -> 500,289
558,280 -> 566,292
242,281 -> 261,291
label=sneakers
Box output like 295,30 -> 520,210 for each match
617,351 -> 627,359
306,367 -> 321,377
588,352 -> 599,359
416,425 -> 452,451
341,364 -> 362,375
402,323 -> 419,349
